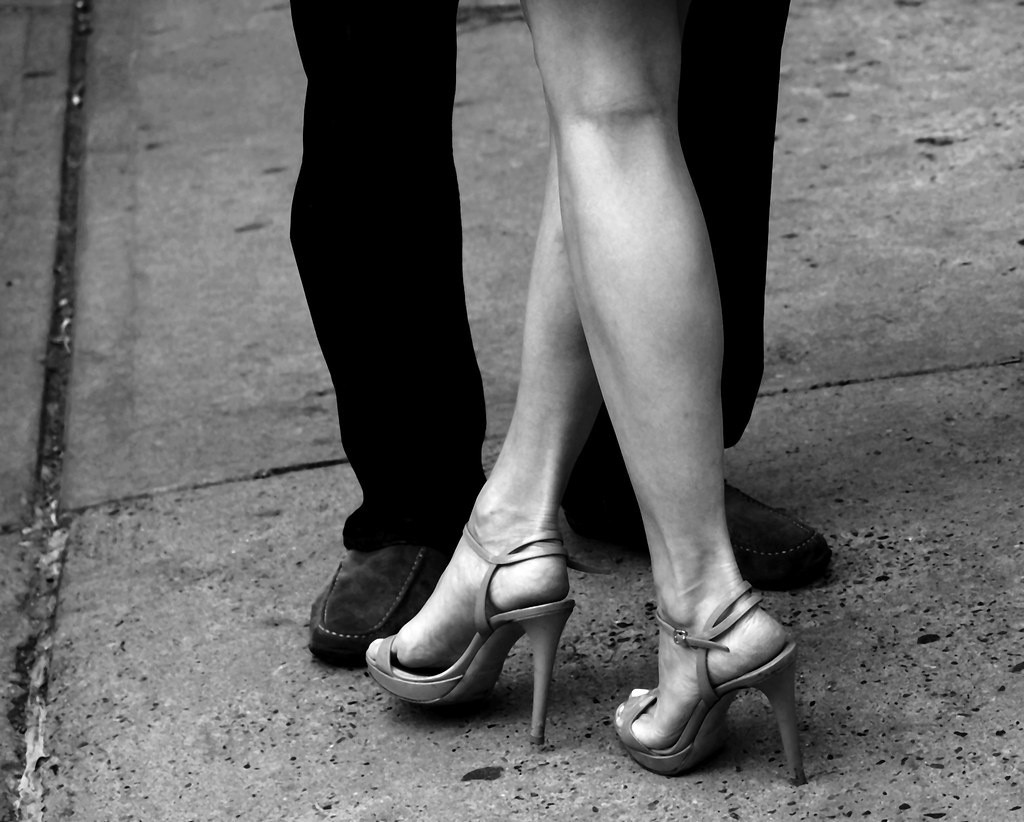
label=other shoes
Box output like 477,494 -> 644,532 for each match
723,477 -> 832,590
309,537 -> 448,669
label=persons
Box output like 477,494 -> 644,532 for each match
289,1 -> 837,669
366,0 -> 808,787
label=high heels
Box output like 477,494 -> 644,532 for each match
614,581 -> 808,786
365,522 -> 575,745
